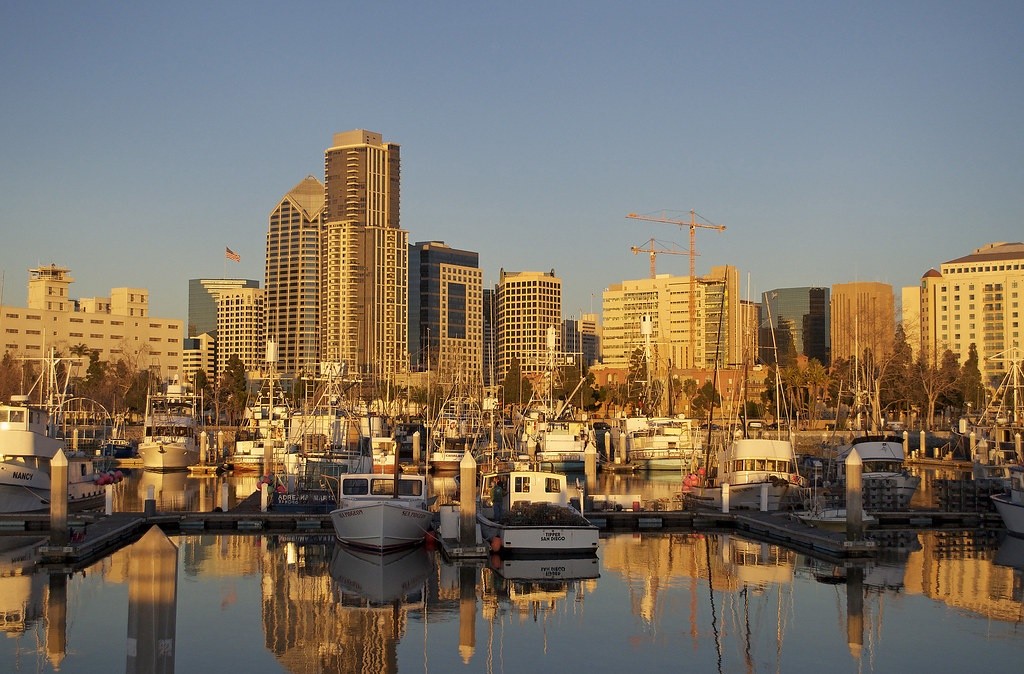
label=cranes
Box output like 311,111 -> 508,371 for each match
624,209 -> 727,367
631,238 -> 702,279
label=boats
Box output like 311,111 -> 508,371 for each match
0,326 -> 108,516
950,347 -> 1024,461
487,548 -> 601,656
139,378 -> 203,471
989,465 -> 1024,538
330,442 -> 432,549
263,363 -> 374,507
329,541 -> 436,642
477,457 -> 600,553
789,505 -> 880,534
101,411 -> 134,461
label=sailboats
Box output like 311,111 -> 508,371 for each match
230,328 -> 499,474
609,310 -> 702,471
512,325 -> 604,474
682,266 -> 808,512
832,528 -> 924,674
823,312 -> 922,510
